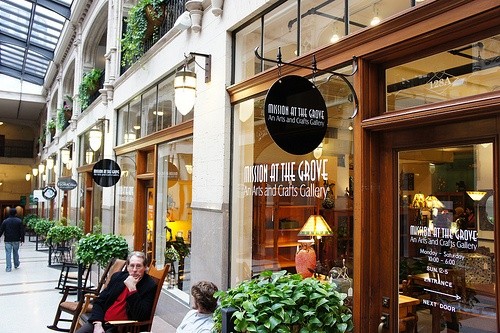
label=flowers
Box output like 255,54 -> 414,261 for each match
48,120 -> 56,128
58,104 -> 70,124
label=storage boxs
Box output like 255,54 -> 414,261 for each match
455,252 -> 491,285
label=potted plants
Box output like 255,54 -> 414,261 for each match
121,0 -> 168,68
79,68 -> 102,111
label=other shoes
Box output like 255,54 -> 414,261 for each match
16,262 -> 20,268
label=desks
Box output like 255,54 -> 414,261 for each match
398,270 -> 496,333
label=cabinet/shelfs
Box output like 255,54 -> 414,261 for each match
252,170 -> 417,268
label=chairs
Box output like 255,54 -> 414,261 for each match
45,257 -> 170,333
430,269 -> 465,333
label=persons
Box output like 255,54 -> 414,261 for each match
0,208 -> 25,272
175,280 -> 218,333
75,250 -> 158,333
454,206 -> 477,230
425,214 -> 462,333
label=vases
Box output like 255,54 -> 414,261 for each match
50,128 -> 55,137
64,110 -> 72,121
322,183 -> 335,209
296,240 -> 317,278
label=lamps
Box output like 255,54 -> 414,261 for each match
467,191 -> 487,230
174,53 -> 211,116
125,129 -> 137,144
85,147 -> 94,164
26,119 -> 110,181
409,192 -> 446,226
66,158 -> 72,170
297,215 -> 335,260
314,142 -> 323,159
